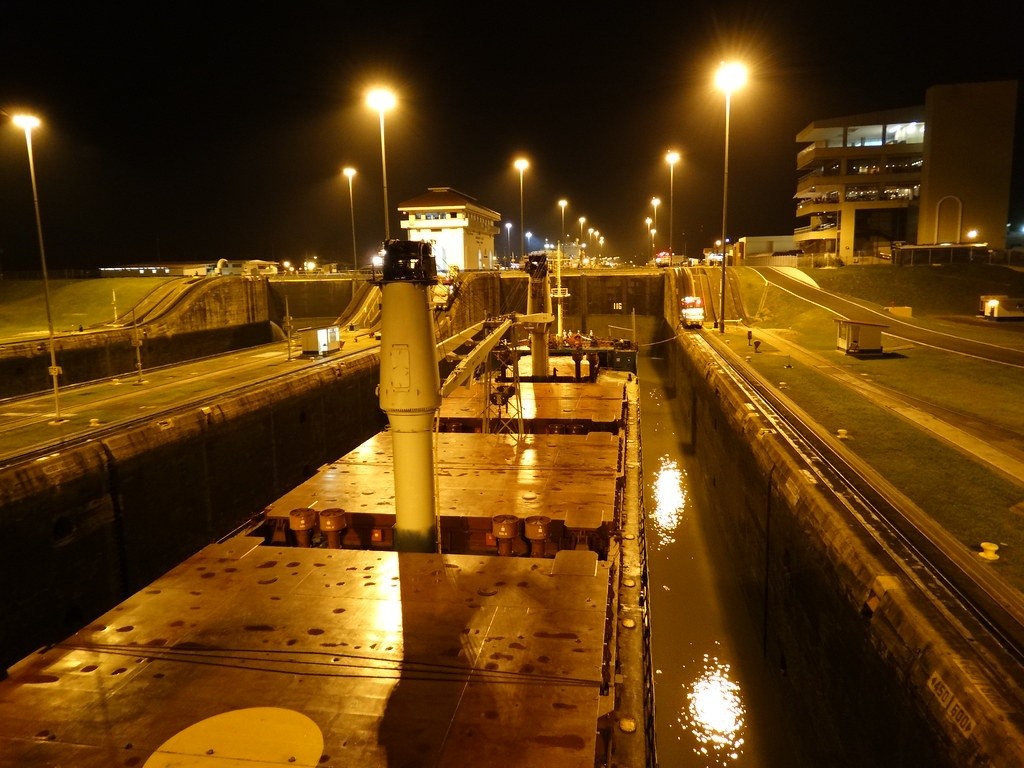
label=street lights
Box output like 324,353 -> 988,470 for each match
557,199 -> 569,240
11,112 -> 62,421
365,86 -> 399,239
504,223 -> 513,260
587,226 -> 605,248
578,217 -> 586,244
650,229 -> 656,257
645,216 -> 651,258
525,232 -> 533,252
651,196 -> 661,229
342,167 -> 361,276
715,62 -> 748,332
515,157 -> 529,261
666,151 -> 680,265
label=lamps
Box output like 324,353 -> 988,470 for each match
754,340 -> 761,353
748,331 -> 752,346
